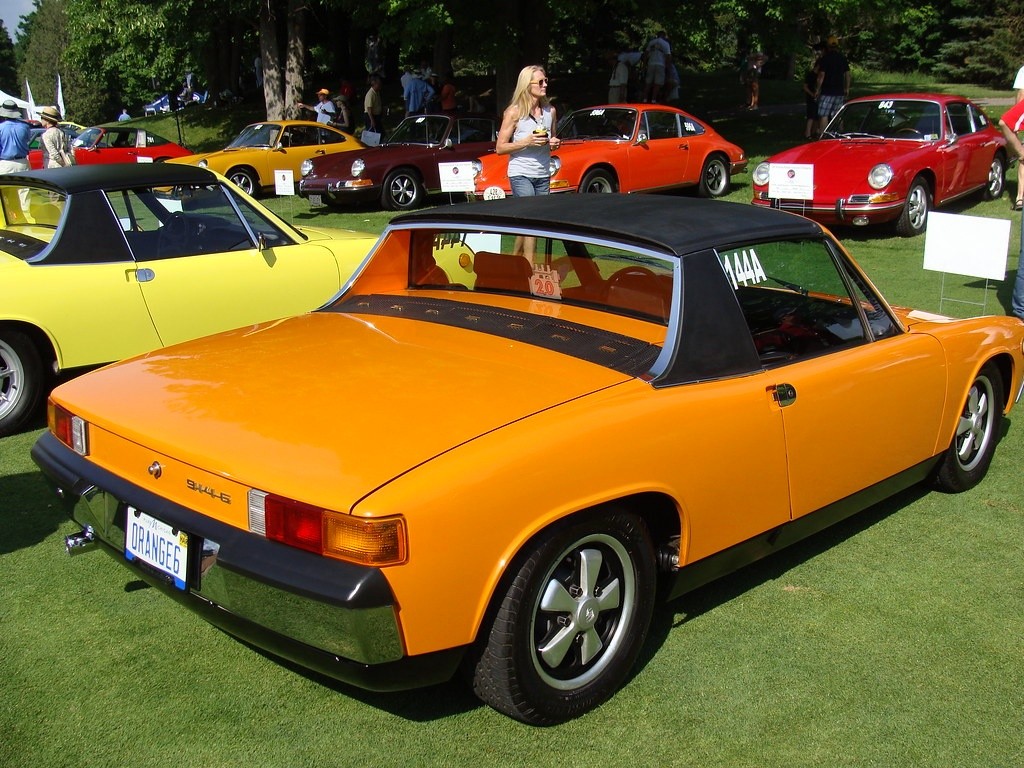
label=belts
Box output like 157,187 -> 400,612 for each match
15,157 -> 22,159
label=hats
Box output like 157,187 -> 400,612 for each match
828,37 -> 838,46
0,99 -> 22,118
36,107 -> 60,123
316,89 -> 329,96
334,95 -> 347,103
605,51 -> 618,59
412,69 -> 423,80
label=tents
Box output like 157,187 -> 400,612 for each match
143,86 -> 203,116
0,90 -> 33,121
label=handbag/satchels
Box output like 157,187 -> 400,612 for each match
361,125 -> 381,147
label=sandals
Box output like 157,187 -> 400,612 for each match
1014,200 -> 1023,210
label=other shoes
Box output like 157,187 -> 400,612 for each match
804,131 -> 813,139
746,105 -> 757,111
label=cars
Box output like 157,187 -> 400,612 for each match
30,192 -> 1024,727
24,121 -> 371,199
751,92 -> 1012,237
294,114 -> 501,211
466,103 -> 748,199
0,164 -> 476,437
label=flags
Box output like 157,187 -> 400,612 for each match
25,81 -> 36,114
55,74 -> 65,120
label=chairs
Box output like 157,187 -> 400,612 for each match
602,267 -> 668,322
469,249 -> 535,292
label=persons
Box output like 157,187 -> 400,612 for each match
255,56 -> 263,87
36,106 -> 72,169
803,57 -> 823,140
365,74 -> 386,143
400,56 -> 458,141
813,35 -> 849,137
118,109 -> 130,122
744,45 -> 762,111
496,65 -> 560,267
298,88 -> 355,136
606,29 -> 680,105
0,100 -> 34,223
998,65 -> 1024,318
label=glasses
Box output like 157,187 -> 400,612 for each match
529,77 -> 549,86
